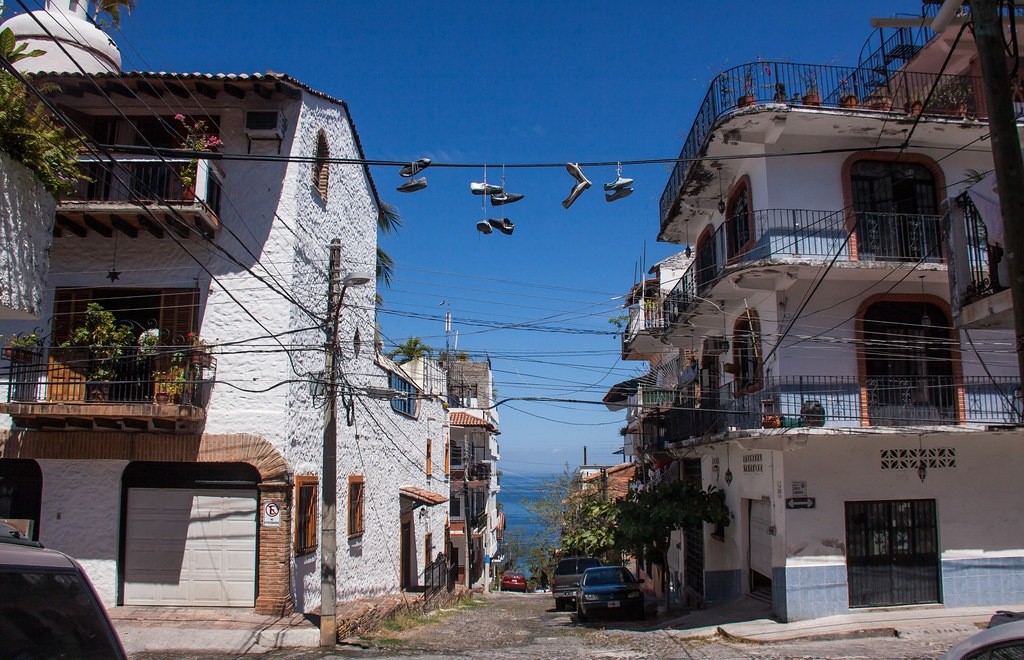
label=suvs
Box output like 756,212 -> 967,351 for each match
553,557 -> 602,612
0,518 -> 128,660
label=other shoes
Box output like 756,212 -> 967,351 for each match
604,177 -> 633,191
396,177 -> 427,192
606,187 -> 633,202
562,182 -> 588,209
566,163 -> 592,186
399,158 -> 431,178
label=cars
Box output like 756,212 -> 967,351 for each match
499,570 -> 529,593
577,566 -> 645,622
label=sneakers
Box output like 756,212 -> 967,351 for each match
471,183 -> 503,195
491,191 -> 524,206
489,218 -> 515,235
477,220 -> 493,234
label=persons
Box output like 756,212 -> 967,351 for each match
540,571 -> 549,593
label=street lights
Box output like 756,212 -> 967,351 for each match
321,272 -> 371,650
